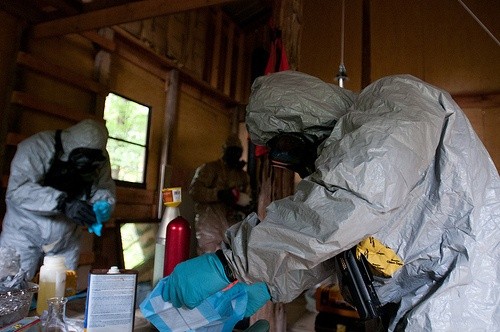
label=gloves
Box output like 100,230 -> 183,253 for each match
57,196 -> 97,227
89,200 -> 111,237
218,186 -> 238,205
161,253 -> 231,311
244,281 -> 272,317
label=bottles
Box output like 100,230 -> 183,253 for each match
42,297 -> 69,332
36,254 -> 66,316
163,215 -> 192,278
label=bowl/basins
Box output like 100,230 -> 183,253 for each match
0,281 -> 39,328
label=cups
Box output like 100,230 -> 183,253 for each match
153,206 -> 181,288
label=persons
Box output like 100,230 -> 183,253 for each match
0,119 -> 115,281
131,72 -> 500,332
